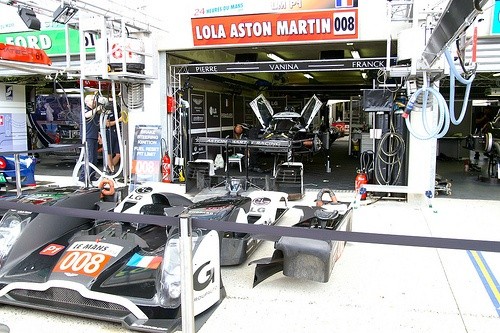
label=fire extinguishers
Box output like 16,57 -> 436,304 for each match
355,167 -> 367,200
161,150 -> 171,183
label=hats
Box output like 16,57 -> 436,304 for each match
98,97 -> 108,104
85,95 -> 94,103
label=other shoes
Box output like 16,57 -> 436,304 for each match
78,176 -> 98,182
107,165 -> 116,175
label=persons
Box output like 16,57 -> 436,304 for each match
105,104 -> 123,175
78,95 -> 101,182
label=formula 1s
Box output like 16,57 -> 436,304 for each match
0,181 -> 290,333
